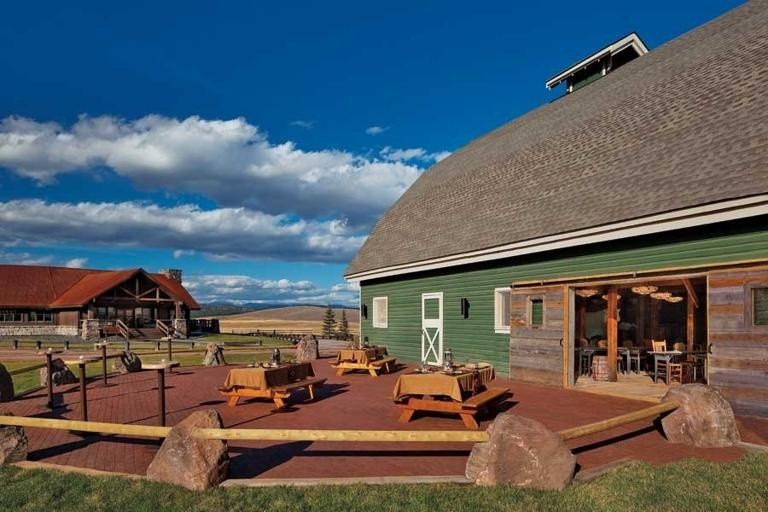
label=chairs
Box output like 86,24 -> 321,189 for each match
646,339 -> 702,384
580,338 -> 633,376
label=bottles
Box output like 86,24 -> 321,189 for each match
272,348 -> 280,363
364,336 -> 369,348
443,348 -> 453,371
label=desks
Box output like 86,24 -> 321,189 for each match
575,346 -> 652,376
647,350 -> 707,384
398,364 -> 494,429
227,362 -> 315,408
335,347 -> 390,377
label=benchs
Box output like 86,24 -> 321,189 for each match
329,357 -> 396,377
385,388 -> 510,429
215,377 -> 328,409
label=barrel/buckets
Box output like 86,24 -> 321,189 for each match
592,355 -> 609,381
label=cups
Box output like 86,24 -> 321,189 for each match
421,361 -> 428,373
289,359 -> 297,364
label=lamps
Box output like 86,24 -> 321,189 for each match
461,297 -> 470,319
361,304 -> 367,319
